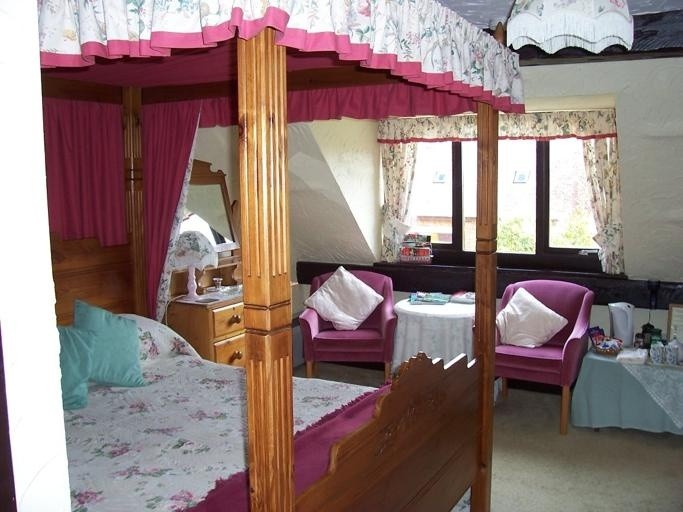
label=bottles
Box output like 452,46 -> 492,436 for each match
634,322 -> 682,366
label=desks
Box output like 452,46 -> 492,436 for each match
570,342 -> 682,436
392,297 -> 498,403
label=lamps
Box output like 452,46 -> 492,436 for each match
505,0 -> 638,58
169,231 -> 219,300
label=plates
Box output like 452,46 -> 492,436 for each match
204,285 -> 225,291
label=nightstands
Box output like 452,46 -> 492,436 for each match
169,284 -> 246,368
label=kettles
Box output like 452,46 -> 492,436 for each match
607,302 -> 634,348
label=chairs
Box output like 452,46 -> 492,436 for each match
496,278 -> 594,435
297,269 -> 397,388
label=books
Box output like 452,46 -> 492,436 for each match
452,289 -> 476,303
409,291 -> 450,304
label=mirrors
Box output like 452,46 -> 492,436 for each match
169,158 -> 240,265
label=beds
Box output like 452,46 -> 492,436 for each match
35,0 -> 527,511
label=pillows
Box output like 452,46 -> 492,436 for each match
58,320 -> 92,411
71,299 -> 151,389
302,264 -> 385,331
110,312 -> 205,368
497,285 -> 567,347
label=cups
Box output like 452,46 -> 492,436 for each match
213,278 -> 223,290
